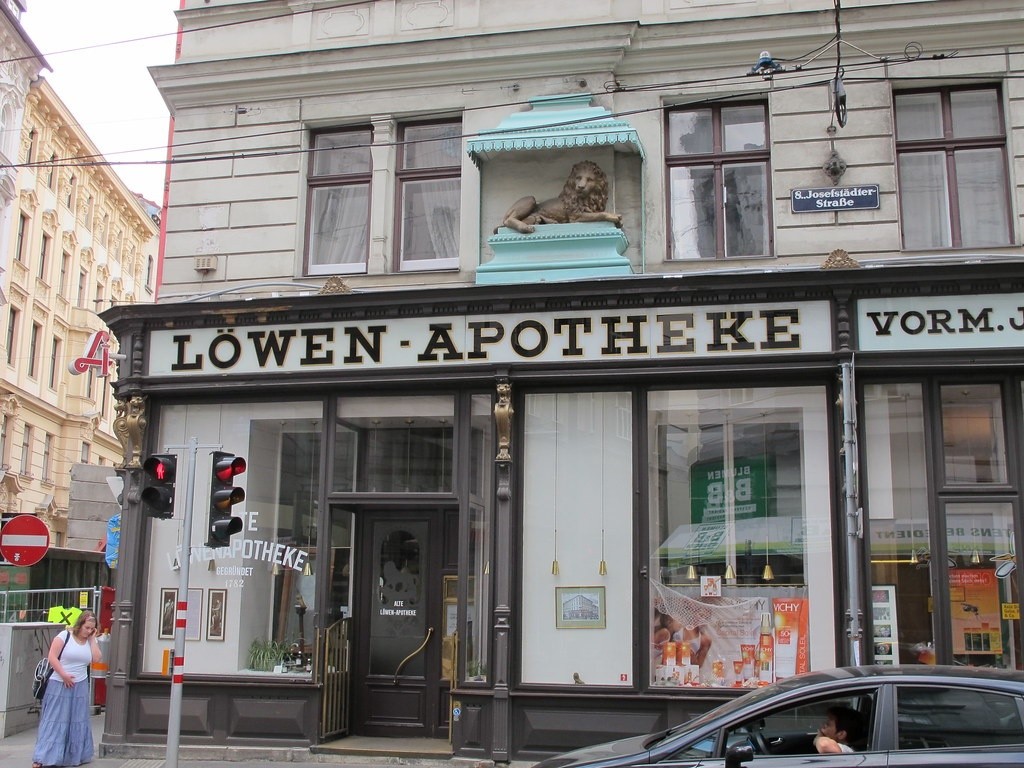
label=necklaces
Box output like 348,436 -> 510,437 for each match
79,641 -> 82,643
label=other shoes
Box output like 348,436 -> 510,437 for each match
31,760 -> 42,768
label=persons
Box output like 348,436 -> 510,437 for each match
671,626 -> 712,670
812,706 -> 864,754
31,610 -> 102,768
653,614 -> 681,667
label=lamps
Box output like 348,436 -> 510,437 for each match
960,385 -> 982,564
552,394 -> 560,576
721,389 -> 736,581
371,418 -> 382,492
404,417 -> 415,492
683,389 -> 701,580
207,405 -> 223,573
303,419 -> 317,576
902,384 -> 918,564
173,405 -> 189,569
597,392 -> 608,576
271,421 -> 286,576
758,388 -> 776,581
483,394 -> 493,576
439,417 -> 448,492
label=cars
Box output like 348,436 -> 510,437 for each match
529,662 -> 1023,767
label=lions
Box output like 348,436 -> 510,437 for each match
492,160 -> 624,234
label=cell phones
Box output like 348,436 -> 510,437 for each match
91,631 -> 94,635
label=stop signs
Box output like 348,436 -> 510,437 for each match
2,516 -> 52,569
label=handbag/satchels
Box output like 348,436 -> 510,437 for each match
32,631 -> 71,699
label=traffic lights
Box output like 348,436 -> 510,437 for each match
203,450 -> 248,550
141,451 -> 178,523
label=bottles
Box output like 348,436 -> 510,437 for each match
306,659 -> 311,673
295,651 -> 303,668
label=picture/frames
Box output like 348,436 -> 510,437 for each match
556,586 -> 606,631
183,588 -> 203,642
157,587 -> 178,641
205,588 -> 227,643
